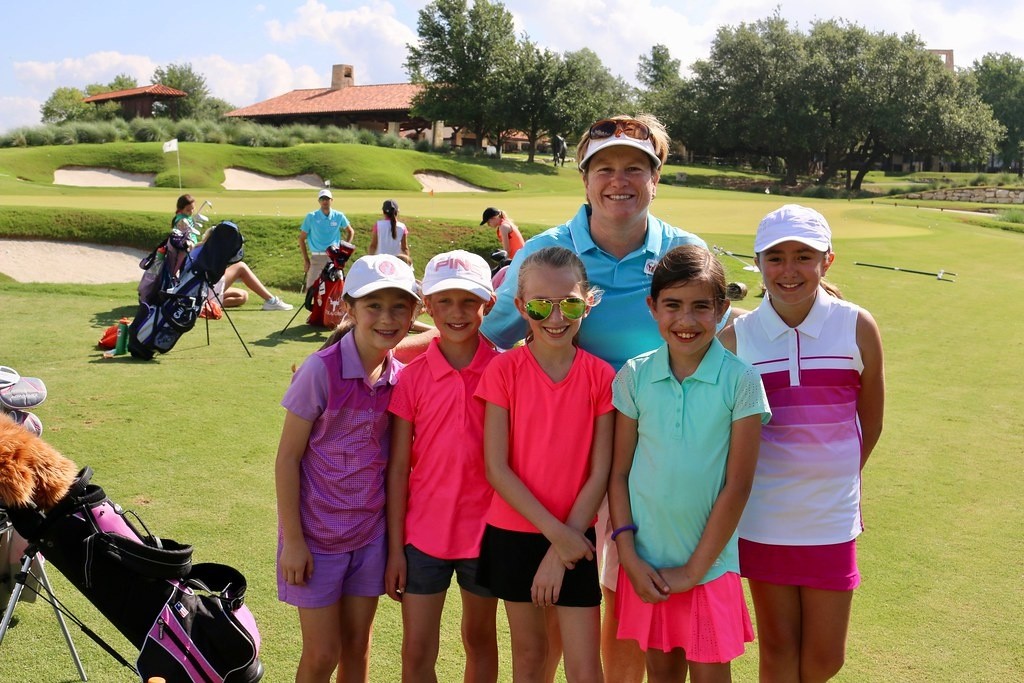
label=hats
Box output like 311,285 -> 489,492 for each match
579,133 -> 661,172
421,248 -> 494,302
480,207 -> 501,226
754,203 -> 832,253
342,253 -> 421,302
382,200 -> 399,214
318,190 -> 332,200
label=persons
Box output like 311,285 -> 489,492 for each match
717,205 -> 884,683
275,255 -> 428,683
476,248 -> 618,683
383,248 -> 510,683
173,110 -> 751,370
592,244 -> 772,683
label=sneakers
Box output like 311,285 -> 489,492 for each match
262,296 -> 294,311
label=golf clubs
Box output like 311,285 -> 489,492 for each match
180,200 -> 214,245
0,365 -> 21,389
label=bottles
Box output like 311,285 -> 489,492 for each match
154,246 -> 166,262
115,317 -> 131,355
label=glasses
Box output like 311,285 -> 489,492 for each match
319,196 -> 331,201
583,119 -> 656,151
521,296 -> 589,321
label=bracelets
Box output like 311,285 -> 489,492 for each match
611,525 -> 636,540
